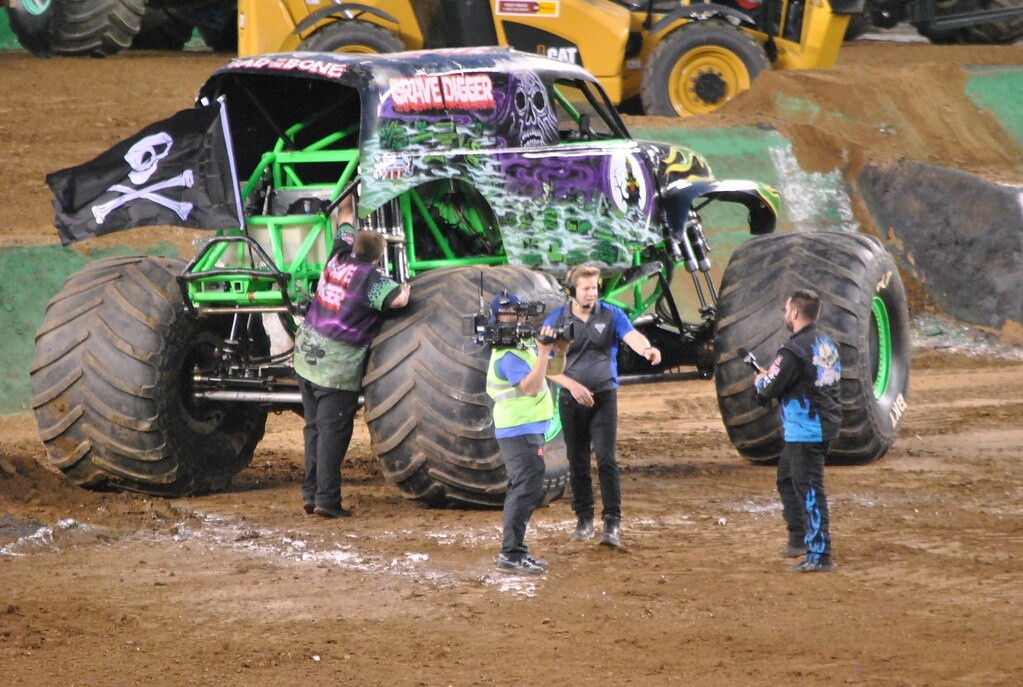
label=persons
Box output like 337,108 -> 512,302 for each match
534,267 -> 661,547
294,181 -> 410,518
486,293 -> 570,575
754,290 -> 842,572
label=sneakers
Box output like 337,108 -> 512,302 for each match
599,514 -> 621,547
495,553 -> 550,575
570,516 -> 595,541
793,560 -> 832,571
778,545 -> 809,557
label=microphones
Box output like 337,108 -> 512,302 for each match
737,348 -> 761,374
573,297 -> 590,308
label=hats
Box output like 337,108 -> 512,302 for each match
487,291 -> 523,325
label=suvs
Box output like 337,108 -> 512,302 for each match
6,1 -> 1022,117
25,43 -> 912,512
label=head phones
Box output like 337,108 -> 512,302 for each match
564,269 -> 603,298
499,289 -> 510,308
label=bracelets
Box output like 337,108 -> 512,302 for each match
643,348 -> 651,356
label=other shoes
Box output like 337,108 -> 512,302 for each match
302,498 -> 316,514
313,505 -> 354,518
